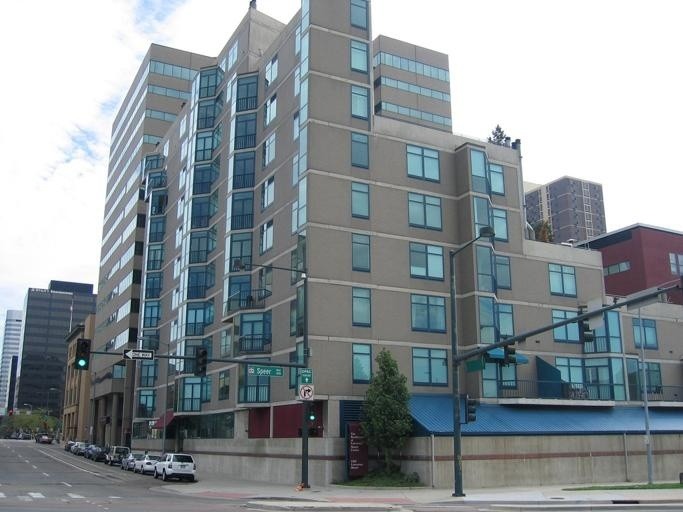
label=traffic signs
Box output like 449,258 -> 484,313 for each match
123,349 -> 154,361
299,367 -> 313,384
247,364 -> 284,377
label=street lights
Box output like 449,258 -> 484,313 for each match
230,257 -> 311,488
23,403 -> 32,416
447,225 -> 496,497
49,387 -> 62,441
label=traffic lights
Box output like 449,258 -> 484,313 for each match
464,392 -> 476,423
503,335 -> 516,364
307,405 -> 316,420
576,310 -> 594,343
73,338 -> 91,370
192,345 -> 208,378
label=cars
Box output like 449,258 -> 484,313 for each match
64,438 -> 196,483
10,431 -> 52,444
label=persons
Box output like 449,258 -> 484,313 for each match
0,424 -> 60,444
125,429 -> 131,452
